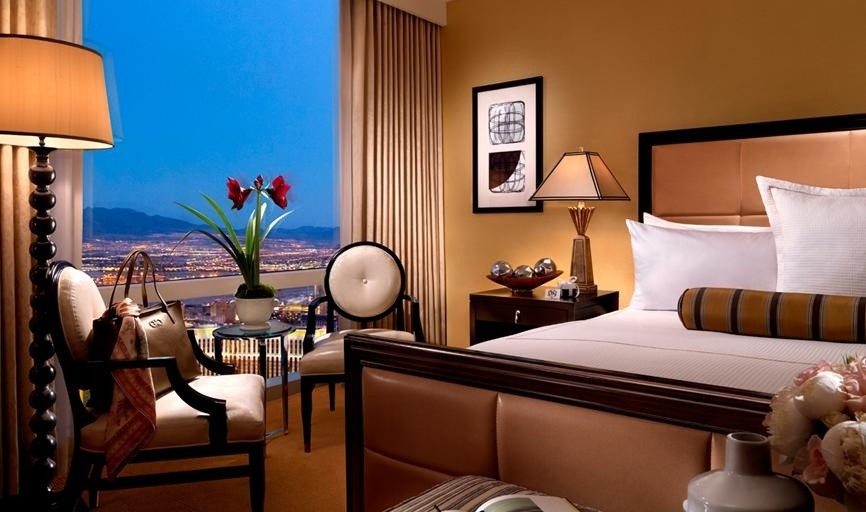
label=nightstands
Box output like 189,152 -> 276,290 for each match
634,113 -> 866,302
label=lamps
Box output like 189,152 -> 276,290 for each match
528,145 -> 631,296
1,37 -> 116,512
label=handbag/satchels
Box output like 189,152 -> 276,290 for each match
84,250 -> 201,412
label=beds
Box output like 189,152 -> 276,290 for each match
346,114 -> 866,512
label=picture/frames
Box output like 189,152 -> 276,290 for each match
472,74 -> 543,214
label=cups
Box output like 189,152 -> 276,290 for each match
558,282 -> 581,297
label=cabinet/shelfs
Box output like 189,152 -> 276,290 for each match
469,279 -> 620,350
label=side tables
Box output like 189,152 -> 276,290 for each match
212,319 -> 296,435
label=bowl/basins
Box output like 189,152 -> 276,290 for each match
486,270 -> 563,294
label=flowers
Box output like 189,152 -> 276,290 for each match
762,354 -> 866,512
179,172 -> 300,299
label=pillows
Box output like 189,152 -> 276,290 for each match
625,218 -> 777,312
643,212 -> 771,232
677,287 -> 866,343
755,175 -> 866,297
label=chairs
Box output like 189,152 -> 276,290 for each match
300,235 -> 426,454
46,261 -> 266,512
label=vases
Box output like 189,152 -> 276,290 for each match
233,296 -> 274,330
676,431 -> 821,512
